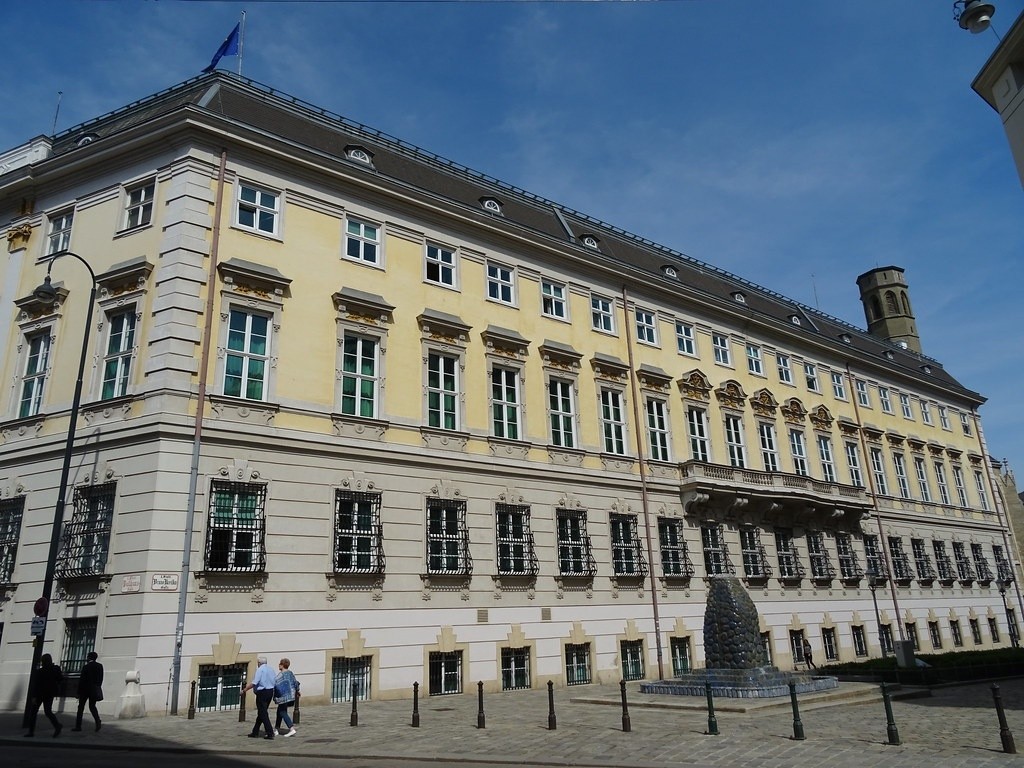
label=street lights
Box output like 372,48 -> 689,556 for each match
995,578 -> 1015,648
24,251 -> 97,726
865,567 -> 886,658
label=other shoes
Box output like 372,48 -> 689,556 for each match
53,723 -> 63,738
71,728 -> 83,732
284,730 -> 296,737
273,729 -> 279,735
95,720 -> 102,733
248,732 -> 259,738
24,734 -> 34,738
264,734 -> 275,739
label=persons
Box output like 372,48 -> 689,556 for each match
22,653 -> 64,739
70,651 -> 104,732
273,658 -> 298,738
802,639 -> 817,670
237,655 -> 276,739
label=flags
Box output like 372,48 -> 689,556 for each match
200,21 -> 239,73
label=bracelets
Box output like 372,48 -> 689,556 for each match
243,690 -> 245,693
